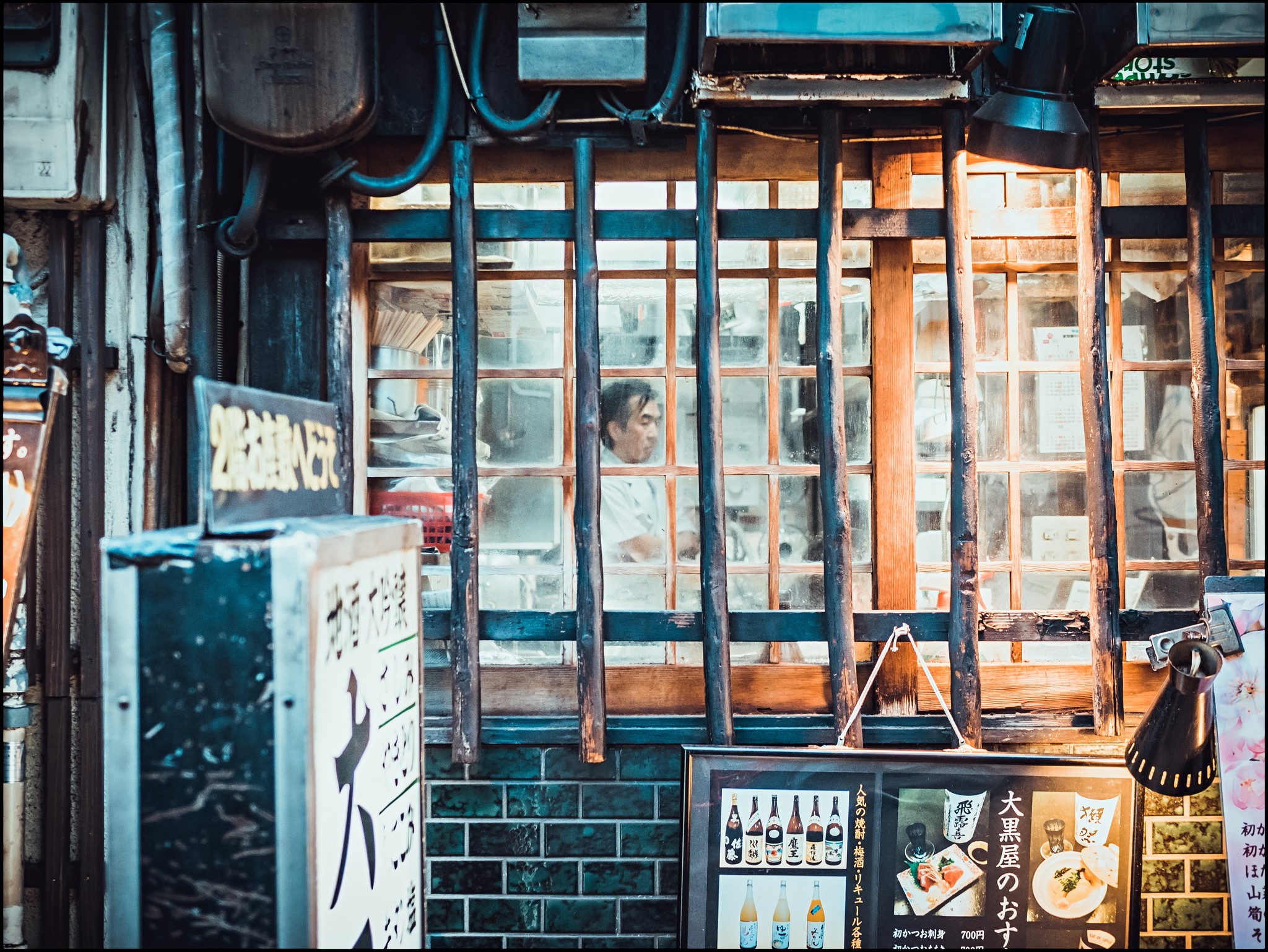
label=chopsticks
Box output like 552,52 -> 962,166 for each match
366,309 -> 445,354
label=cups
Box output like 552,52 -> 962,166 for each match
369,346 -> 420,417
905,822 -> 927,859
1043,818 -> 1066,856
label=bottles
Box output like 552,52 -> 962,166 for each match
806,795 -> 823,866
745,794 -> 763,865
772,880 -> 790,949
765,794 -> 783,866
786,795 -> 804,866
943,788 -> 987,844
739,879 -> 758,949
724,793 -> 743,864
806,880 -> 825,950
825,796 -> 843,866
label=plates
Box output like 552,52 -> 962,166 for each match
905,840 -> 935,863
968,841 -> 988,865
1040,839 -> 1073,860
1031,851 -> 1108,919
896,844 -> 984,916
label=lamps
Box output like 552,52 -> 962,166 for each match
965,4 -> 1107,169
1124,638 -> 1224,797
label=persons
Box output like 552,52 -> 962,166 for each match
599,379 -> 700,564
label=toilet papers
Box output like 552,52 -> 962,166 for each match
757,526 -> 808,564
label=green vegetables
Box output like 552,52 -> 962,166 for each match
903,856 -> 954,890
1053,866 -> 1086,893
